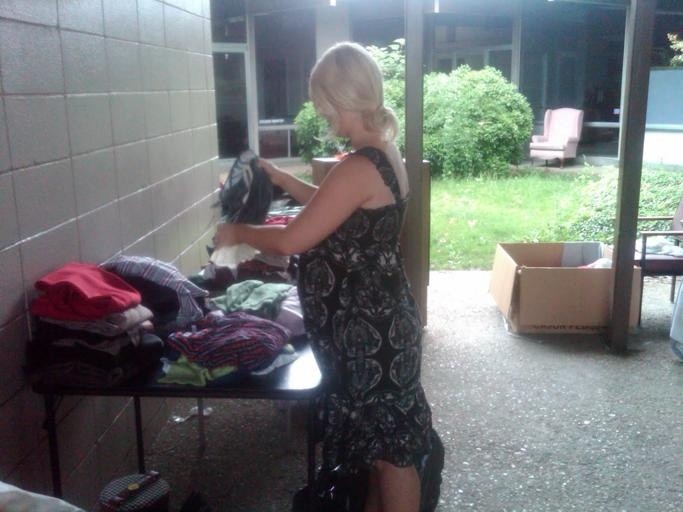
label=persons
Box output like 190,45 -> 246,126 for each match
212,40 -> 432,511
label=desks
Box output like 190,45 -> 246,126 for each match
28,285 -> 331,508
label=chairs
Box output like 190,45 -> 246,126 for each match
612,196 -> 683,327
528,107 -> 584,169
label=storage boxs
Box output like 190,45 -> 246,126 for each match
490,241 -> 642,334
